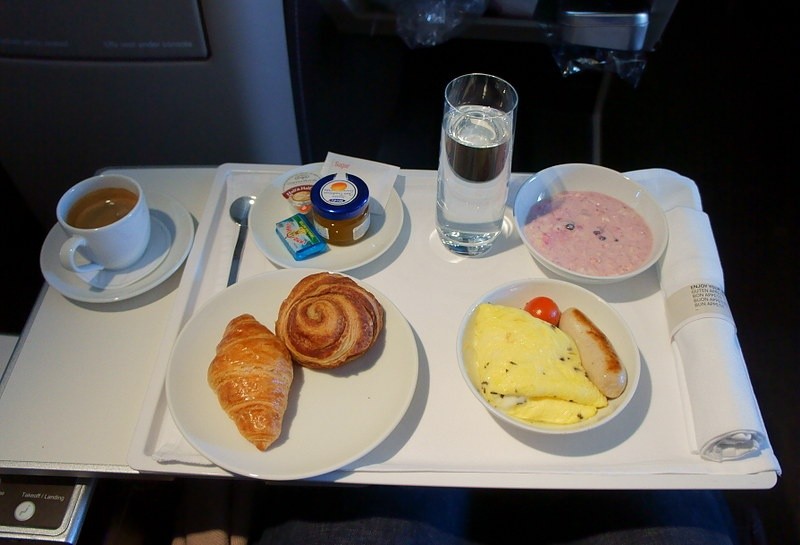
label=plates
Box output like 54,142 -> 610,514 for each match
40,192 -> 195,303
248,162 -> 404,272
165,268 -> 420,481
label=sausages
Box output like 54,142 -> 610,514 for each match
559,307 -> 628,398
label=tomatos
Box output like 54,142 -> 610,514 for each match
524,296 -> 561,327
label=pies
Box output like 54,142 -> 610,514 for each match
474,303 -> 609,424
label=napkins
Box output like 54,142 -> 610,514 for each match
653,206 -> 769,464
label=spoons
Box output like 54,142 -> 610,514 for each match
227,195 -> 255,286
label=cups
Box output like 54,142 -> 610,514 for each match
436,73 -> 519,258
56,174 -> 151,272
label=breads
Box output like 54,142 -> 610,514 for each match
207,313 -> 294,451
275,272 -> 384,368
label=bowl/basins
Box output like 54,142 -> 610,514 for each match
455,278 -> 642,434
513,162 -> 670,283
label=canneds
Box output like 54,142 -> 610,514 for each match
310,173 -> 370,246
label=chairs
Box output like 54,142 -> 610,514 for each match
0,0 -> 320,340
